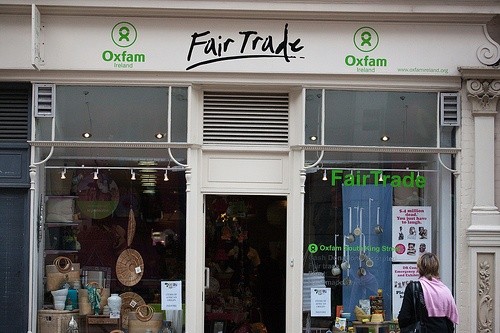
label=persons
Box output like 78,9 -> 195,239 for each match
397,252 -> 459,333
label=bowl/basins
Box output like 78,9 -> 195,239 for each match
340,313 -> 351,319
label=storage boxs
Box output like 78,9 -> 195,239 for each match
369,295 -> 384,313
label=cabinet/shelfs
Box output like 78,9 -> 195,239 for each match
44,193 -> 78,262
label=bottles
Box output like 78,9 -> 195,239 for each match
158,320 -> 177,333
65,296 -> 73,310
107,294 -> 122,314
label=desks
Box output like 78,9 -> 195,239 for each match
353,321 -> 392,333
86,315 -> 120,333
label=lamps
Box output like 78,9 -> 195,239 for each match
164,170 -> 169,181
131,169 -> 136,180
60,168 -> 66,179
379,173 -> 383,181
93,169 -> 98,180
322,170 -> 327,181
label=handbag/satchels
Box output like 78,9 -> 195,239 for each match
400,320 -> 421,333
